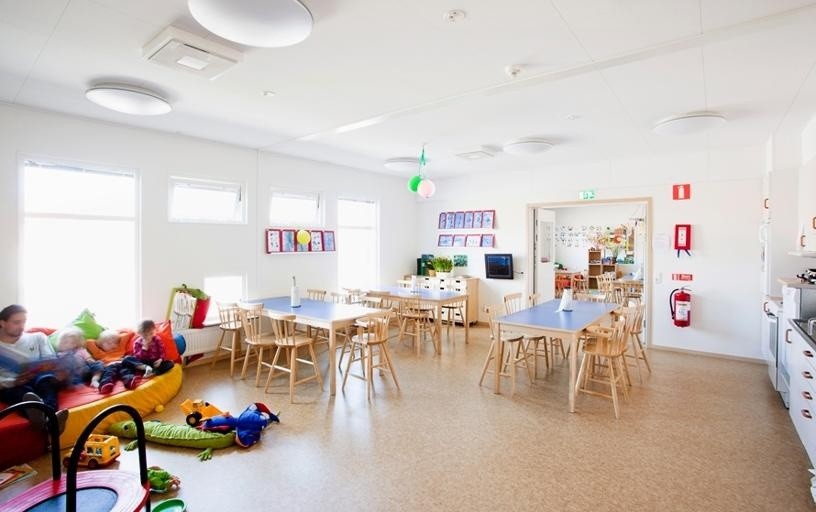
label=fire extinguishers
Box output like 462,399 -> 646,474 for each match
669,284 -> 693,329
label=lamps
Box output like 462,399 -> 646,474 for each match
384,156 -> 428,175
651,110 -> 728,144
83,83 -> 172,120
500,139 -> 554,158
185,1 -> 315,51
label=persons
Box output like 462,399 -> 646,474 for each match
55,326 -> 104,388
0,304 -> 69,438
133,320 -> 174,379
86,327 -> 142,395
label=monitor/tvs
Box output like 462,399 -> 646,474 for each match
485,254 -> 513,279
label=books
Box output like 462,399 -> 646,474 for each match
0,462 -> 38,489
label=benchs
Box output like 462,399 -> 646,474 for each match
0,322 -> 183,470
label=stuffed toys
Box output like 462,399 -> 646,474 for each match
107,399 -> 280,462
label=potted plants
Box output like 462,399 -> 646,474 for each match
431,256 -> 453,273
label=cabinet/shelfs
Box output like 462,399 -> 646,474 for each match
761,169 -> 806,299
795,156 -> 816,257
586,249 -> 618,290
757,292 -> 815,474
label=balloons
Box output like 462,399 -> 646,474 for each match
418,179 -> 436,199
407,175 -> 421,193
296,230 -> 310,245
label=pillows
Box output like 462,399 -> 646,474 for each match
136,318 -> 182,366
70,306 -> 104,341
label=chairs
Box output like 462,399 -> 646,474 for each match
478,270 -> 653,420
212,270 -> 481,406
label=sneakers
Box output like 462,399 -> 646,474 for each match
23,392 -> 45,431
127,376 -> 141,390
45,408 -> 68,436
98,379 -> 114,394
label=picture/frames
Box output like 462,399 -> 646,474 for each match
264,227 -> 336,254
437,209 -> 496,249
